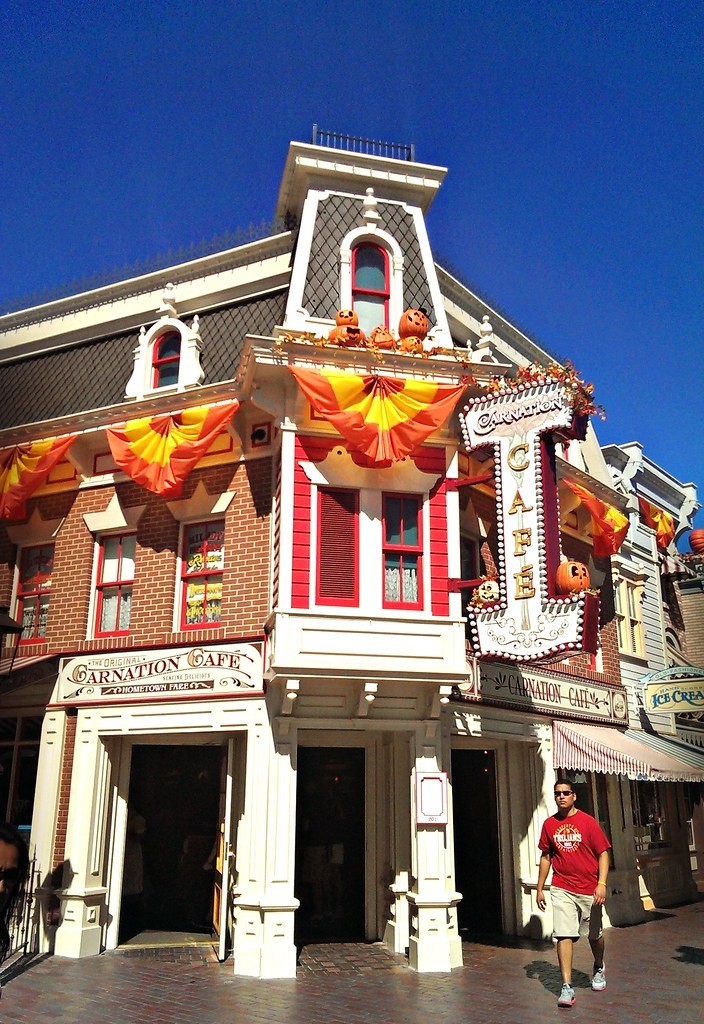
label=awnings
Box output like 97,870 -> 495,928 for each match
552,720 -> 704,782
624,729 -> 704,771
666,642 -> 700,679
659,553 -> 697,583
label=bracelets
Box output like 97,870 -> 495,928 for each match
598,881 -> 607,886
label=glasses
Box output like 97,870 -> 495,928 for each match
554,790 -> 573,796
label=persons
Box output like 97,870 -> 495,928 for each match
536,780 -> 612,1006
121,804 -> 145,929
0,820 -> 29,961
191,842 -> 217,931
303,775 -> 345,927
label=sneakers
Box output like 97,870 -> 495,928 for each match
557,983 -> 576,1007
592,961 -> 606,991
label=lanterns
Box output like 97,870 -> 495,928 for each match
329,309 -> 428,354
556,561 -> 590,595
